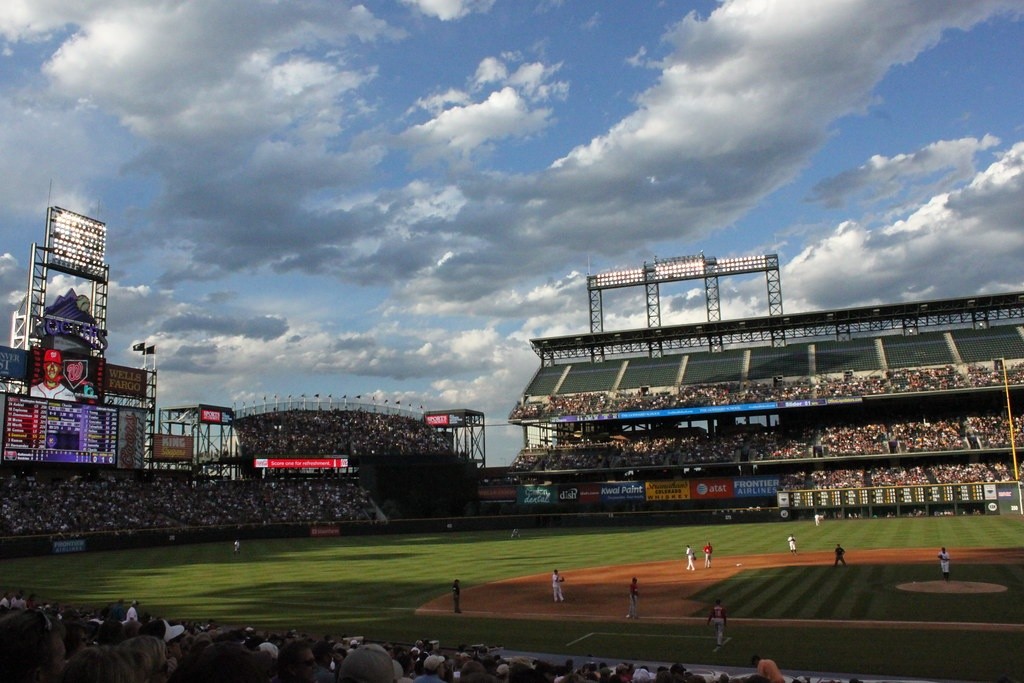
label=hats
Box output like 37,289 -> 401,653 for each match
258,642 -> 280,659
162,619 -> 184,642
340,643 -> 393,683
424,655 -> 445,671
496,664 -> 509,676
44,350 -> 62,364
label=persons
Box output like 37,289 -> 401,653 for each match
787,534 -> 799,556
685,544 -> 696,571
1,590 -> 876,683
702,542 -> 713,568
814,512 -> 820,526
510,528 -> 522,538
551,569 -> 566,602
937,546 -> 950,585
451,579 -> 463,613
833,544 -> 848,567
507,357 -> 1024,518
233,538 -> 241,556
625,576 -> 641,621
1,390 -> 456,544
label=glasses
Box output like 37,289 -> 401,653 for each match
301,659 -> 317,667
